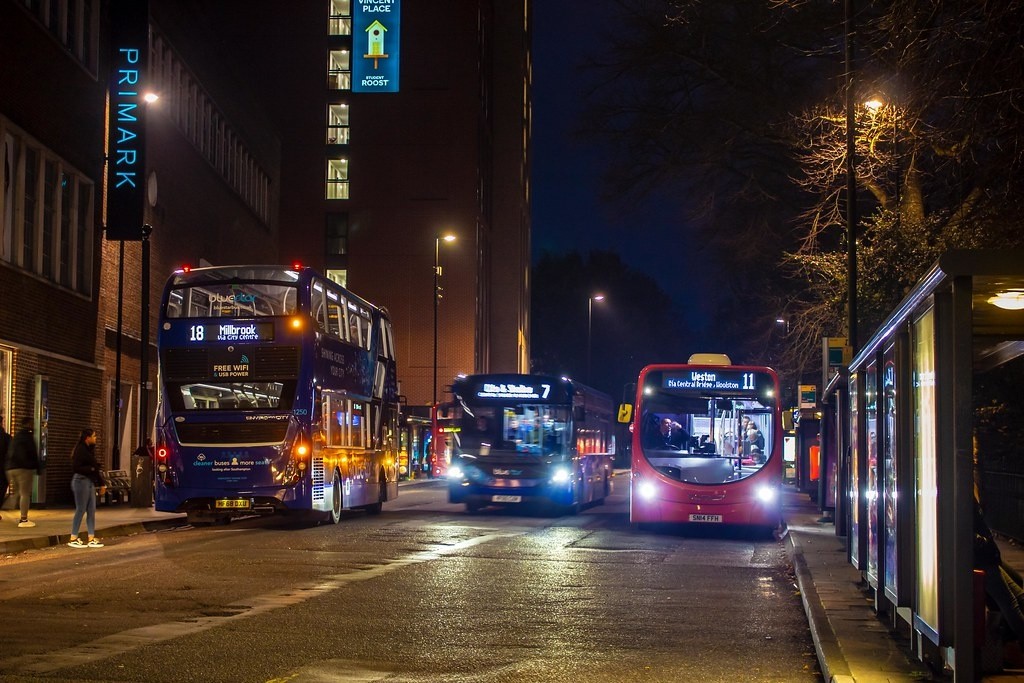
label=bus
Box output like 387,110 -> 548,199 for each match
617,354 -> 795,539
154,264 -> 407,528
432,402 -> 487,480
441,372 -> 618,516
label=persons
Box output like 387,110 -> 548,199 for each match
351,326 -> 365,346
651,418 -> 690,450
68,428 -> 105,548
726,415 -> 765,466
508,414 -> 550,444
318,315 -> 334,334
426,442 -> 434,479
6,417 -> 38,528
974,496 -> 1024,643
133,437 -> 155,456
472,416 -> 498,445
0,415 -> 11,519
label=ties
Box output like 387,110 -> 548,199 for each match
665,433 -> 669,438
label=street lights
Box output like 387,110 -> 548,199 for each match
864,92 -> 900,223
777,319 -> 789,334
433,236 -> 456,404
587,296 -> 604,387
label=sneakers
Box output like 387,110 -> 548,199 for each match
88,538 -> 104,547
18,518 -> 36,527
68,538 -> 87,548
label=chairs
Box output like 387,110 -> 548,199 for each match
724,432 -> 733,443
